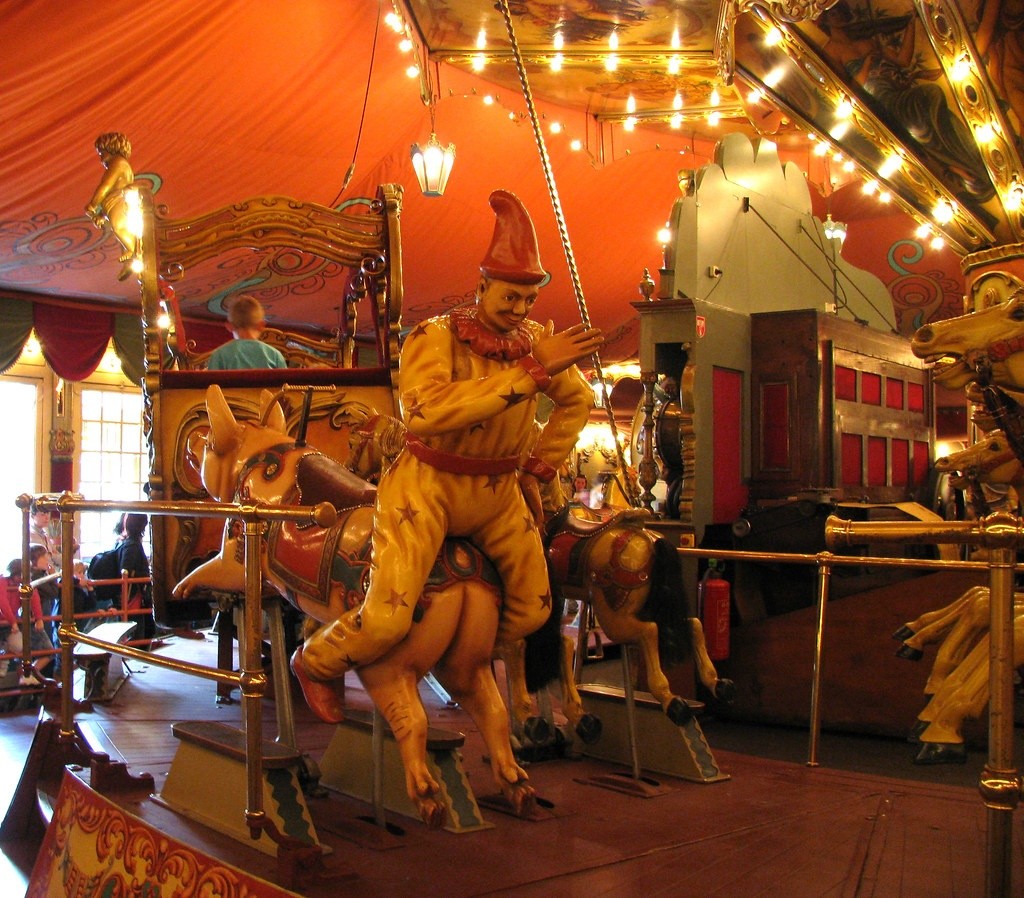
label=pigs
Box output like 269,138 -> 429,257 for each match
168,382 -> 544,829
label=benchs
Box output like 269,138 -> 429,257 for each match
73,622 -> 137,700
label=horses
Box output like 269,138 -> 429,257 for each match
492,476 -> 742,749
892,288 -> 1024,765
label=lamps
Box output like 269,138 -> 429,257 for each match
823,213 -> 848,253
410,69 -> 456,197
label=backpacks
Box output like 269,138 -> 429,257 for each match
88,539 -> 142,601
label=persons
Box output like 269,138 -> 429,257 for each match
288,190 -> 604,728
29,543 -> 59,633
574,475 -> 587,492
51,558 -> 97,675
116,513 -> 156,641
84,132 -> 141,281
209,295 -> 287,369
0,559 -> 55,671
28,510 -> 62,568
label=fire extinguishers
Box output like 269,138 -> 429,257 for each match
697,558 -> 730,661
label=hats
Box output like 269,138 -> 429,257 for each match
30,544 -> 47,560
6,559 -> 21,570
73,559 -> 84,572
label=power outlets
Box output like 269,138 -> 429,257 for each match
825,303 -> 837,313
710,266 -> 719,278
